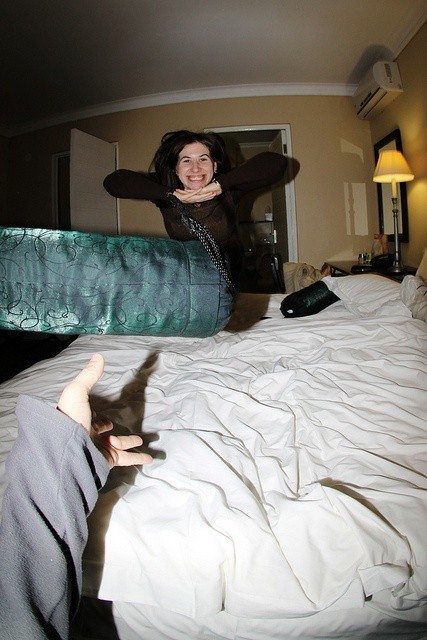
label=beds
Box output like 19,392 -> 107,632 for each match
1,273 -> 426,640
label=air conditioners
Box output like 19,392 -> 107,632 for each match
352,61 -> 403,121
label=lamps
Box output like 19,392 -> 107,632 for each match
373,150 -> 415,274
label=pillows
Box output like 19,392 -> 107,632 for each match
323,275 -> 401,315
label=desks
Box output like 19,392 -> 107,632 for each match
326,260 -> 363,276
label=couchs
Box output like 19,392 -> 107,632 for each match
283,261 -> 326,293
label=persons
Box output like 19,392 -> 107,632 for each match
0,353 -> 153,639
103,130 -> 288,293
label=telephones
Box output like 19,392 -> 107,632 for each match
370,253 -> 395,267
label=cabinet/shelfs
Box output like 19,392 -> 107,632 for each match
240,217 -> 273,243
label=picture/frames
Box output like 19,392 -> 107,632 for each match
374,128 -> 409,243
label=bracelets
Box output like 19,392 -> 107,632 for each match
212,178 -> 221,188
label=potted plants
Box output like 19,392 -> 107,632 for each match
264,206 -> 272,221
